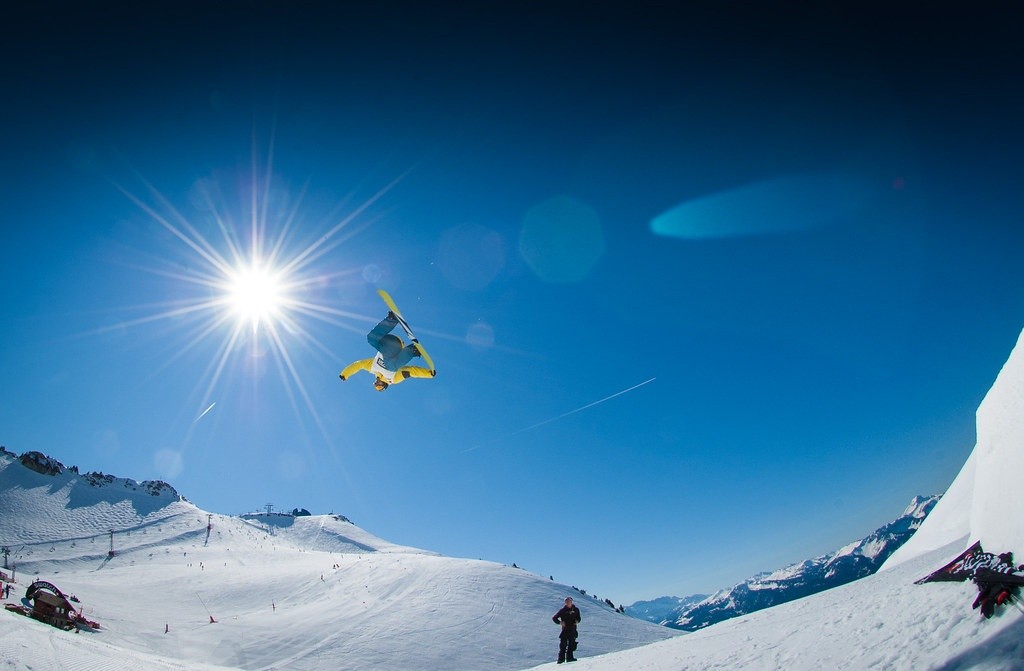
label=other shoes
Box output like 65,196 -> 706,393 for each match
557,661 -> 562,664
414,347 -> 420,356
567,658 -> 577,661
387,311 -> 395,320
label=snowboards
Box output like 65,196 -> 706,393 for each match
375,287 -> 436,375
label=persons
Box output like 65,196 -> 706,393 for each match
340,312 -> 436,392
5,583 -> 9,599
552,597 -> 581,664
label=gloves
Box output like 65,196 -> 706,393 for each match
339,375 -> 345,381
431,370 -> 436,376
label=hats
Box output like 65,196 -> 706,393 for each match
376,383 -> 388,391
564,596 -> 573,603
